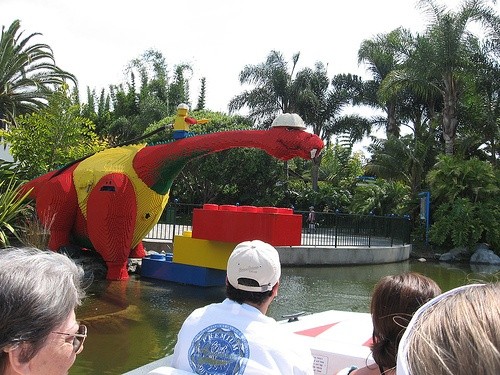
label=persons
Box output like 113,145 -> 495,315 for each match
170,239 -> 315,375
0,246 -> 88,375
395,282 -> 500,375
306,206 -> 317,234
335,272 -> 441,375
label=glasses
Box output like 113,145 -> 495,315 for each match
51,326 -> 87,354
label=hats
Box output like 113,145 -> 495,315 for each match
309,206 -> 314,209
227,240 -> 281,293
